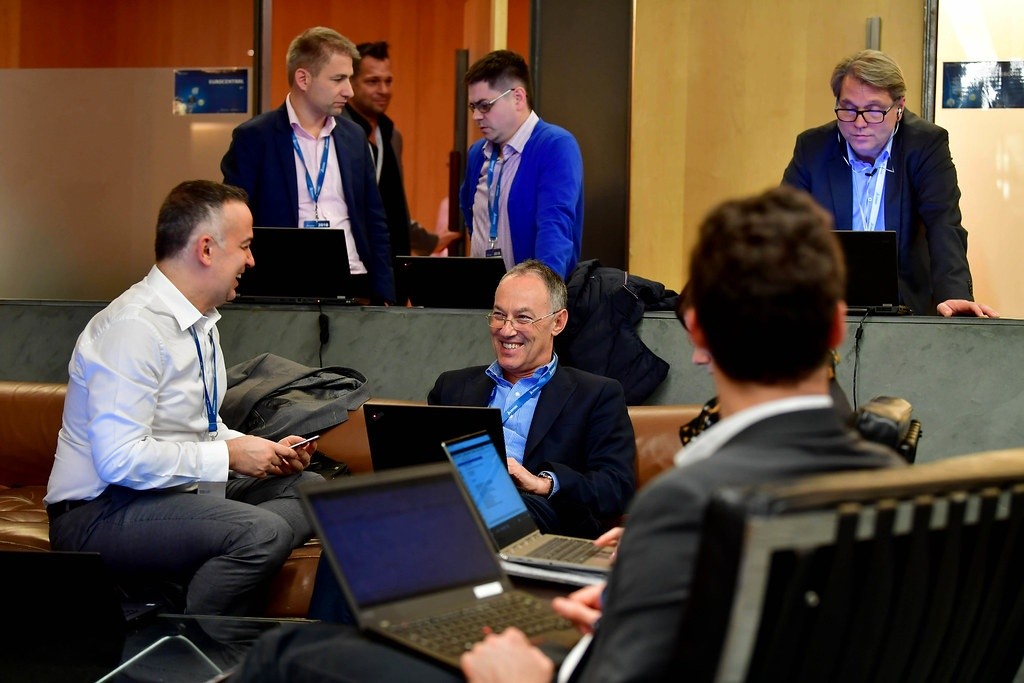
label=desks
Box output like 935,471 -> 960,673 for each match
0,299 -> 1024,465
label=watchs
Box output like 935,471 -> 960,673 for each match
540,473 -> 554,497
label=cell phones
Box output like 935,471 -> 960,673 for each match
289,435 -> 319,449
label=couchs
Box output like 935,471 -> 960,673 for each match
1,381 -> 719,616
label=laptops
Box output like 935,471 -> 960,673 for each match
239,226 -> 358,306
396,256 -> 507,309
296,404 -> 616,672
829,230 -> 913,315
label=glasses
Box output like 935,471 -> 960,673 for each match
468,89 -> 528,114
485,308 -> 562,333
833,95 -> 897,124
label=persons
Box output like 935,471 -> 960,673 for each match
458,186 -> 910,683
780,49 -> 999,318
427,260 -> 638,540
339,41 -> 465,307
42,180 -> 327,617
459,50 -> 584,285
220,27 -> 394,307
592,277 -> 720,565
